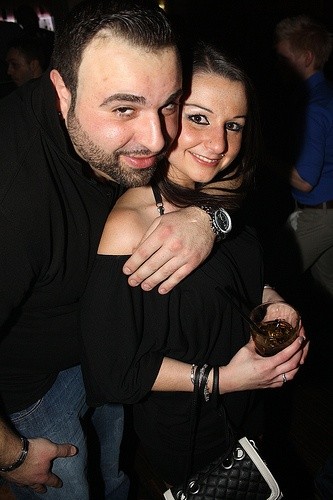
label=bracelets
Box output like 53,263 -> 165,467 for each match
0,436 -> 30,473
191,364 -> 211,402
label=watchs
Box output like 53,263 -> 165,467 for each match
197,200 -> 232,243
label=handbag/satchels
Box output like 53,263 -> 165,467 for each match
163,365 -> 284,500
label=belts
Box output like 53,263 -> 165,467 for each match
297,201 -> 333,210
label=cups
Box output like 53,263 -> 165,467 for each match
249,301 -> 302,357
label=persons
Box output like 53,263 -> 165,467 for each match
272,14 -> 333,304
0,1 -> 257,500
78,41 -> 311,500
6,29 -> 48,87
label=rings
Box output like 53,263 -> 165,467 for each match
283,373 -> 287,383
